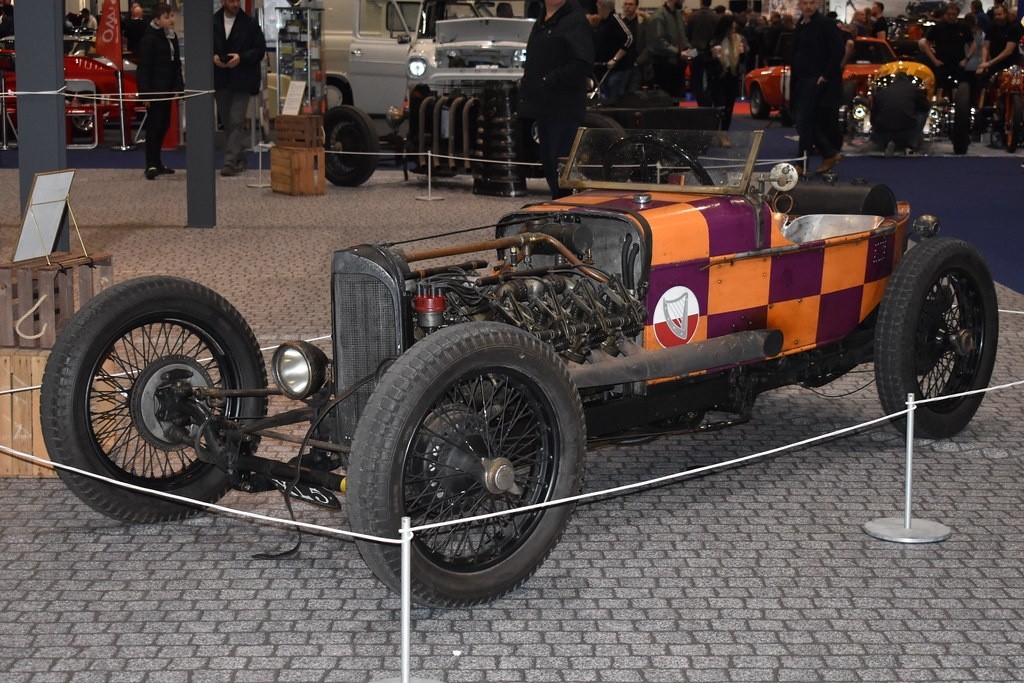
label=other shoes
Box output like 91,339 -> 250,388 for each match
817,153 -> 841,174
157,165 -> 176,174
719,130 -> 732,147
794,165 -> 807,176
234,167 -> 245,172
220,165 -> 235,176
144,165 -> 158,179
552,188 -> 577,200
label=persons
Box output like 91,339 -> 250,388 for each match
0,4 -> 14,37
212,0 -> 268,176
123,0 -> 186,180
523,0 -> 616,199
79,9 -> 98,34
596,0 -> 1024,157
790,0 -> 847,177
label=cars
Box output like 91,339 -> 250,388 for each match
266,0 -> 500,154
744,37 -> 897,127
